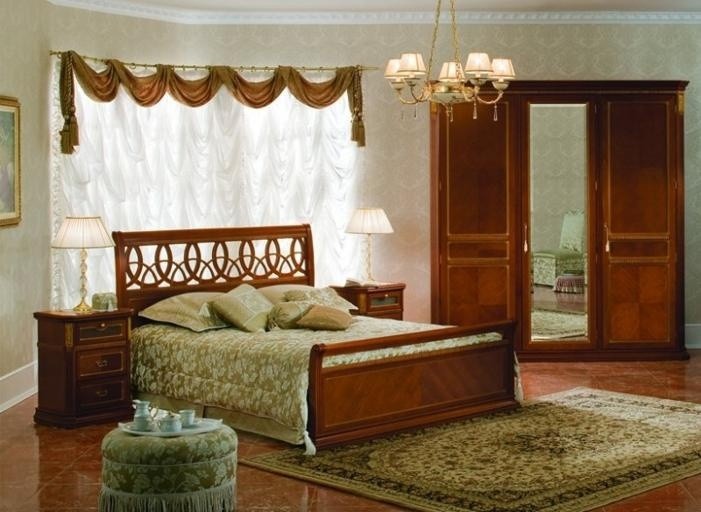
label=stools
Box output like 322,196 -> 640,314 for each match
554,274 -> 586,294
100,419 -> 237,511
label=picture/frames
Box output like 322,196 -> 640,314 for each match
0,95 -> 22,228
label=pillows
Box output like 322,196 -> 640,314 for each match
209,282 -> 275,332
265,301 -> 311,331
282,285 -> 360,313
258,280 -> 315,303
138,291 -> 231,334
294,303 -> 352,329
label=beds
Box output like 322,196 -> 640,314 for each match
109,222 -> 527,455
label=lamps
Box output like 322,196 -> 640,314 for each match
383,0 -> 517,125
51,214 -> 117,314
345,207 -> 394,284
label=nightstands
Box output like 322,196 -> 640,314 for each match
29,308 -> 137,428
331,283 -> 405,322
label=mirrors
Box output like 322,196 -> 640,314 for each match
522,101 -> 597,350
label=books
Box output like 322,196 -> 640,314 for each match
345,276 -> 399,287
563,270 -> 585,276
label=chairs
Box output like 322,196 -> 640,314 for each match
534,208 -> 588,287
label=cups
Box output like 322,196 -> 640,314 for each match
155,413 -> 184,434
179,409 -> 196,427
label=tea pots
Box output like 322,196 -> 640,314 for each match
129,399 -> 157,432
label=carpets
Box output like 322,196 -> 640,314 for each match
236,382 -> 701,512
